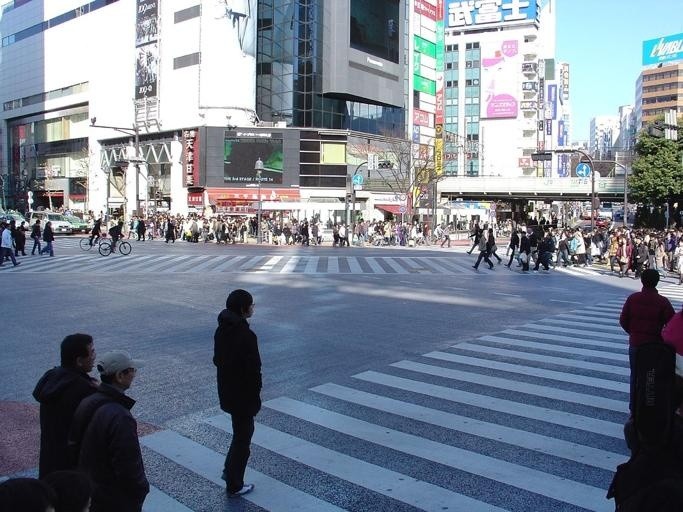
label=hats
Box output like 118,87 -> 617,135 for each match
97,350 -> 145,375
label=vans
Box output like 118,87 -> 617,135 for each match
28,212 -> 71,235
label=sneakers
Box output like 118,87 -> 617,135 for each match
227,484 -> 254,498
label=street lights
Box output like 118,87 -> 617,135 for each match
580,159 -> 627,228
254,157 -> 264,244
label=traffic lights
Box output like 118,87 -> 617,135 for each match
531,153 -> 551,161
377,162 -> 393,170
114,161 -> 129,168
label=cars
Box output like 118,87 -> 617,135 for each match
0,212 -> 31,232
582,202 -> 629,228
63,215 -> 90,234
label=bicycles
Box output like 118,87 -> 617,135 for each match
78,233 -> 111,250
98,237 -> 131,256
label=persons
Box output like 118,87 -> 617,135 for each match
327,208 -> 683,283
39,468 -> 94,512
616,266 -> 671,416
0,207 -> 324,266
66,349 -> 151,512
34,329 -> 97,478
0,475 -> 55,511
211,287 -> 263,502
604,372 -> 683,512
